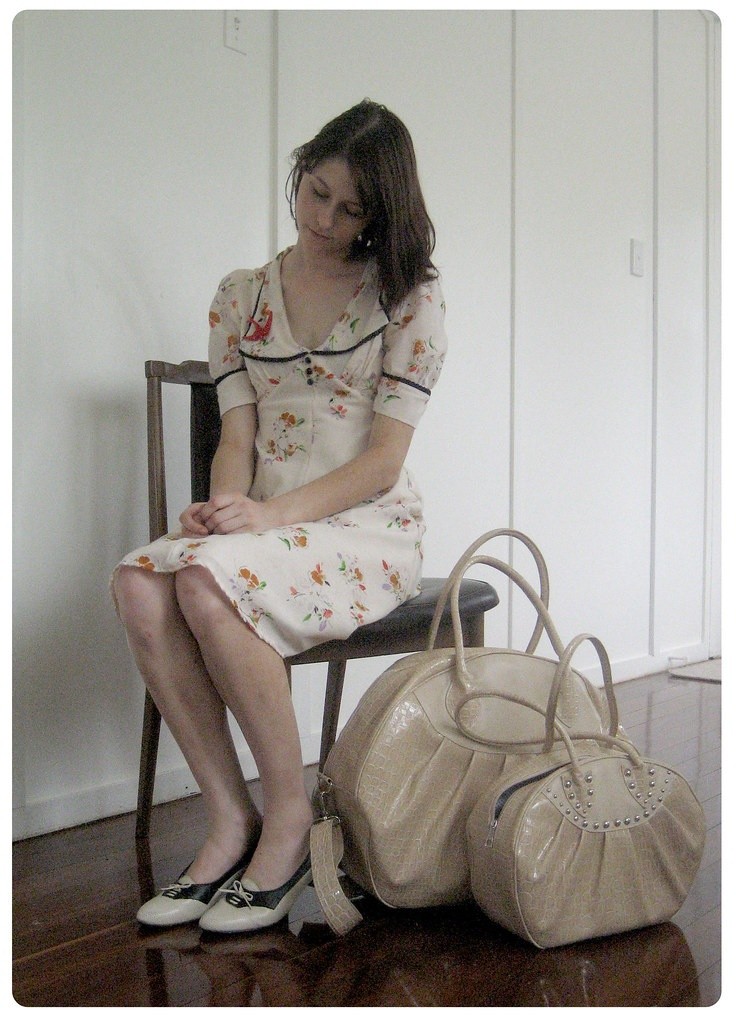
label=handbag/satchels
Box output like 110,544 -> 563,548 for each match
309,528 -> 707,947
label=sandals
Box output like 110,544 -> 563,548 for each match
134,815 -> 313,932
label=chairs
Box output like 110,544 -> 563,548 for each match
132,358 -> 501,840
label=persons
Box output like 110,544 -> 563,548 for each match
112,96 -> 454,934
